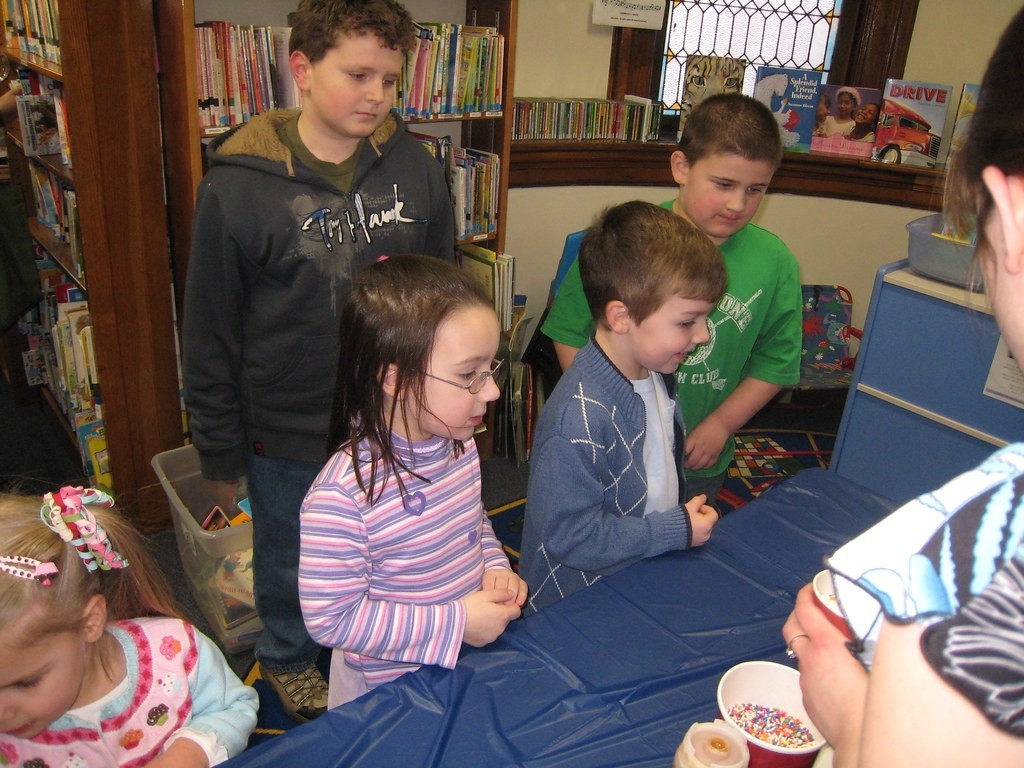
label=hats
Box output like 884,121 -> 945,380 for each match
835,87 -> 860,110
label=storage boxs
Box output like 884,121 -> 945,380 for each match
150,444 -> 265,654
906,213 -> 984,293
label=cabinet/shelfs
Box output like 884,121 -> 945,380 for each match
0,0 -> 183,536
829,257 -> 1024,504
157,1 -> 518,459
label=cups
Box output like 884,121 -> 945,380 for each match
717,661 -> 827,768
812,569 -> 853,639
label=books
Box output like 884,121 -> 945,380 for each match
456,244 -> 545,468
983,334 -> 1024,411
511,93 -> 662,144
0,0 -> 114,495
392,21 -> 504,119
195,19 -> 303,127
405,128 -> 501,242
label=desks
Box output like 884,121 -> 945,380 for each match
209,467 -> 903,767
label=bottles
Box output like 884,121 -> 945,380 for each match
674,719 -> 750,768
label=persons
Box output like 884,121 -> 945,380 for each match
541,92 -> 802,508
816,86 -> 862,137
515,196 -> 730,625
814,92 -> 830,137
781,0 -> 1024,767
183,0 -> 458,724
846,102 -> 879,142
0,486 -> 262,768
294,257 -> 529,714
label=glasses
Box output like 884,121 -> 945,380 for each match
398,359 -> 504,394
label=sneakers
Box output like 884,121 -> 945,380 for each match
258,661 -> 329,723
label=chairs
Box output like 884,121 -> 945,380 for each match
762,284 -> 863,411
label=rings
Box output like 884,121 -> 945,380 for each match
785,634 -> 811,660
